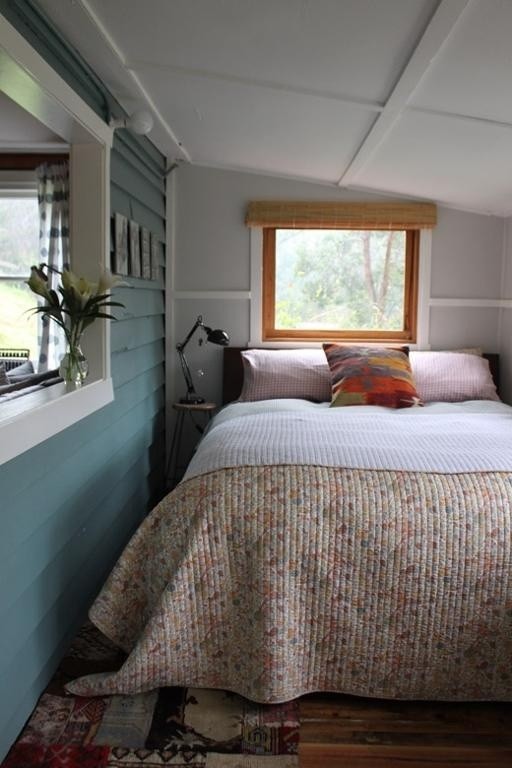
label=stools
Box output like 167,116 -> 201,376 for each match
167,404 -> 217,482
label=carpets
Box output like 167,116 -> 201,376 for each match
0,616 -> 300,768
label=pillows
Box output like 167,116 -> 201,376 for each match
233,344 -> 503,407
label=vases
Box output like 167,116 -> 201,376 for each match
58,330 -> 89,385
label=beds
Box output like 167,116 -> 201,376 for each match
63,348 -> 510,704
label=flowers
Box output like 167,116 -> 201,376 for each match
21,258 -> 126,382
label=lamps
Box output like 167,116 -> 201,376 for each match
109,109 -> 155,135
174,311 -> 230,405
